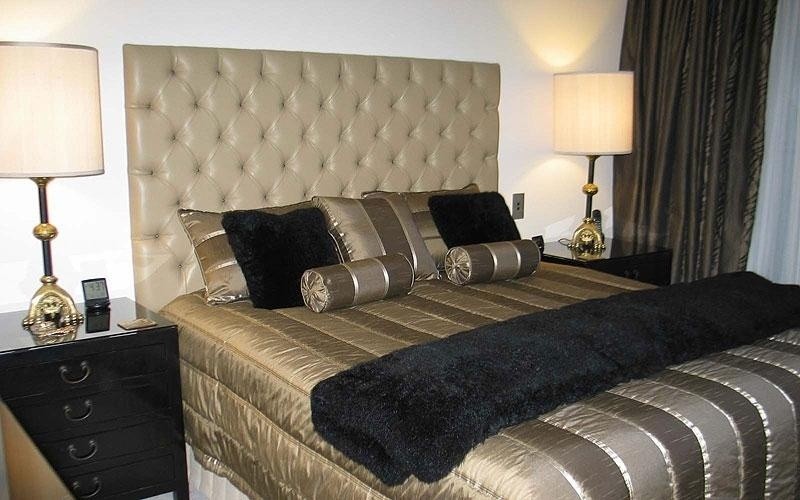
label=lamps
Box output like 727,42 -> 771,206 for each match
0,40 -> 106,332
551,70 -> 636,248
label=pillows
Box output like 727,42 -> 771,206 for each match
311,192 -> 443,281
301,254 -> 415,311
427,192 -> 522,247
222,206 -> 332,312
365,182 -> 480,269
444,240 -> 539,285
177,200 -> 320,304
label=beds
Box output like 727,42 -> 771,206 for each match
120,42 -> 800,500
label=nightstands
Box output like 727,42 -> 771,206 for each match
0,296 -> 190,500
538,240 -> 675,287
